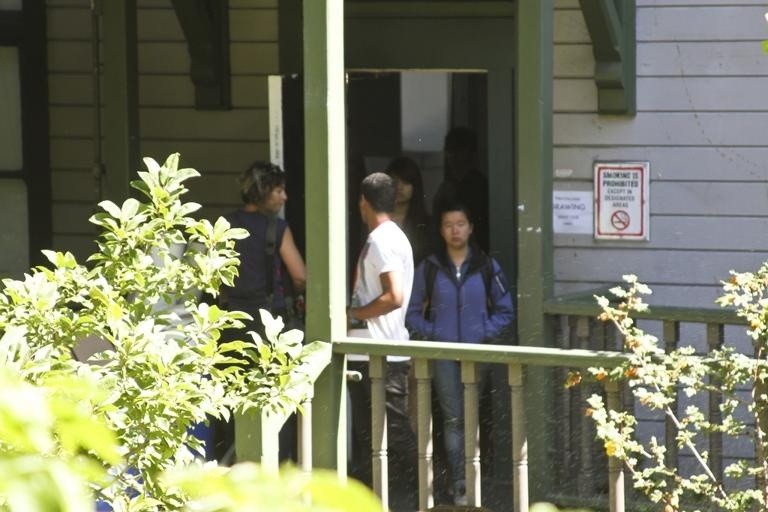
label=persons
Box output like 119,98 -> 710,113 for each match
384,156 -> 433,269
202,161 -> 306,463
424,127 -> 489,256
346,173 -> 420,511
404,203 -> 518,506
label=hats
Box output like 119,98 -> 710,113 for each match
236,162 -> 278,193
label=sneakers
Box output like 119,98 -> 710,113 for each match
454,479 -> 467,507
